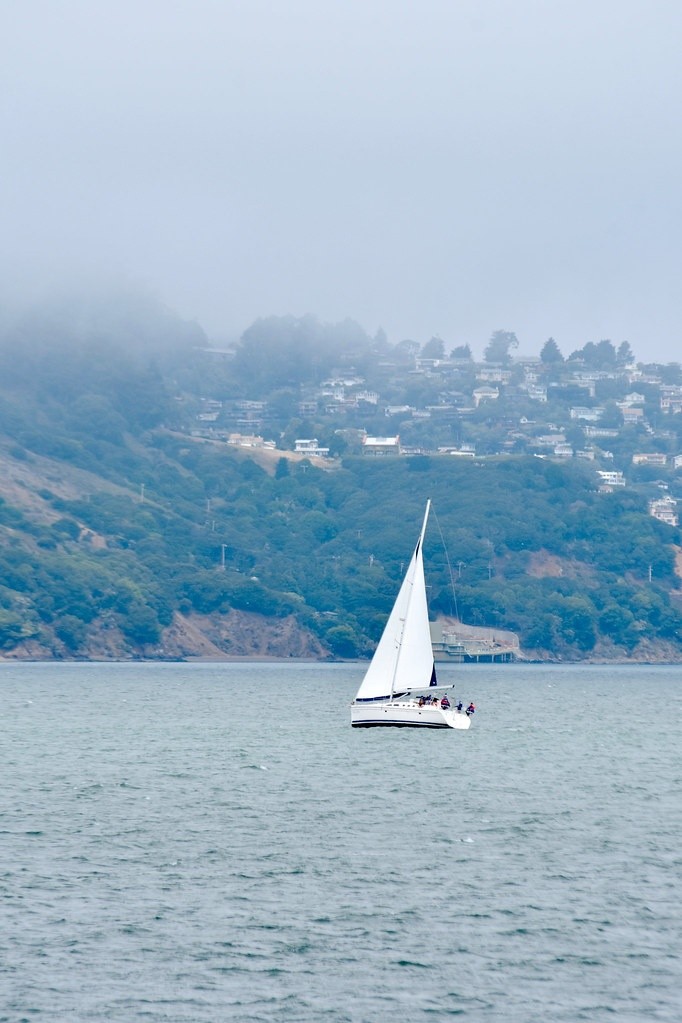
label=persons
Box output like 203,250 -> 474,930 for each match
418,693 -> 451,710
456,702 -> 463,714
465,703 -> 475,716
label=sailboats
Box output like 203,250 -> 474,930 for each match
349,493 -> 479,731
